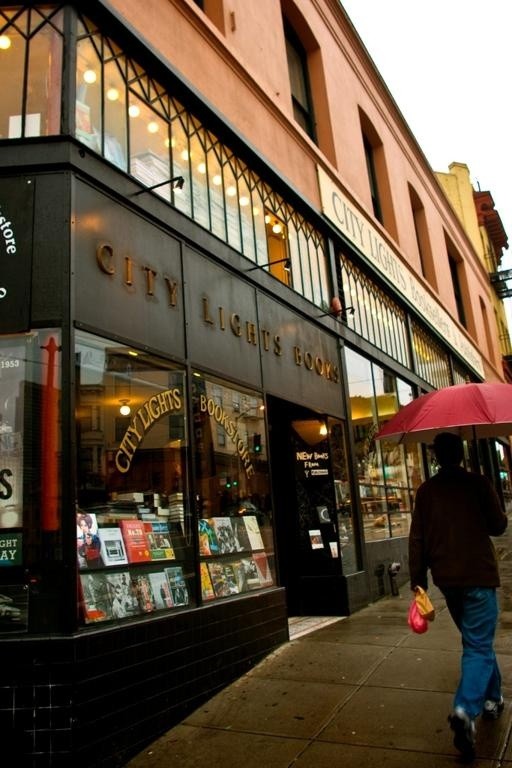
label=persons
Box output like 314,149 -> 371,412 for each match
409,432 -> 505,758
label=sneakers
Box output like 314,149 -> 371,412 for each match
483,695 -> 505,721
447,705 -> 477,753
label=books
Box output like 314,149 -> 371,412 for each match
74,491 -> 273,626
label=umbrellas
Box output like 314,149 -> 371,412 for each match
375,379 -> 512,466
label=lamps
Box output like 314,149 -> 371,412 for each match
317,306 -> 355,319
246,256 -> 294,275
130,175 -> 185,196
